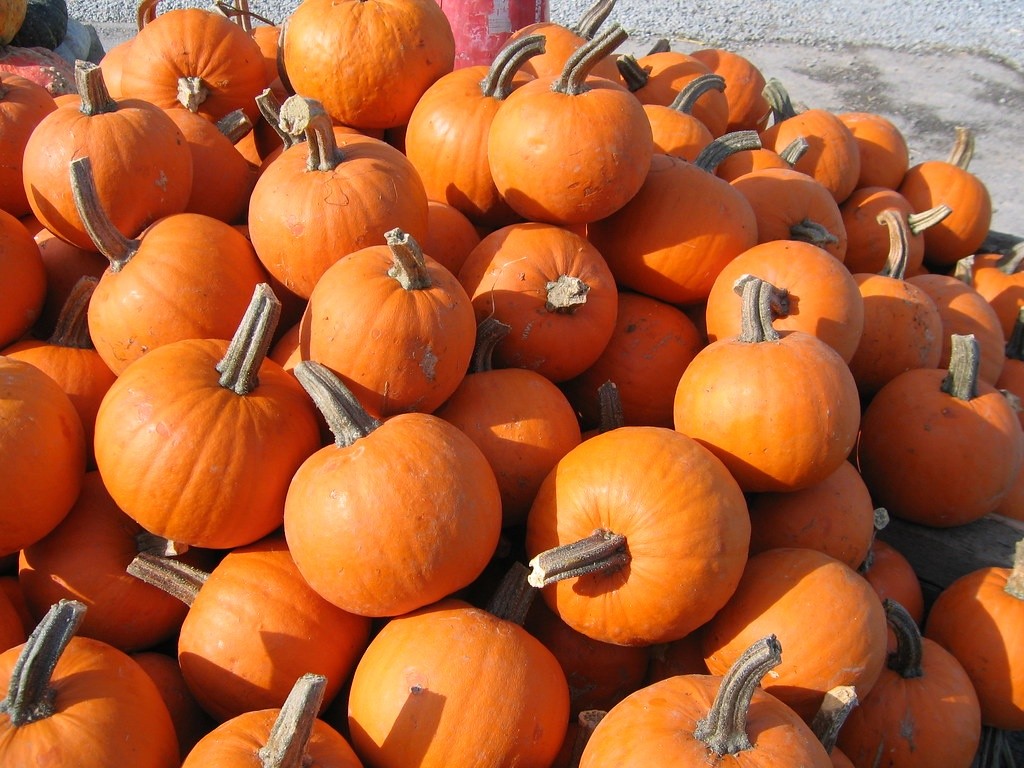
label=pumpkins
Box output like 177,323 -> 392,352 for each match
0,1 -> 1024,768
